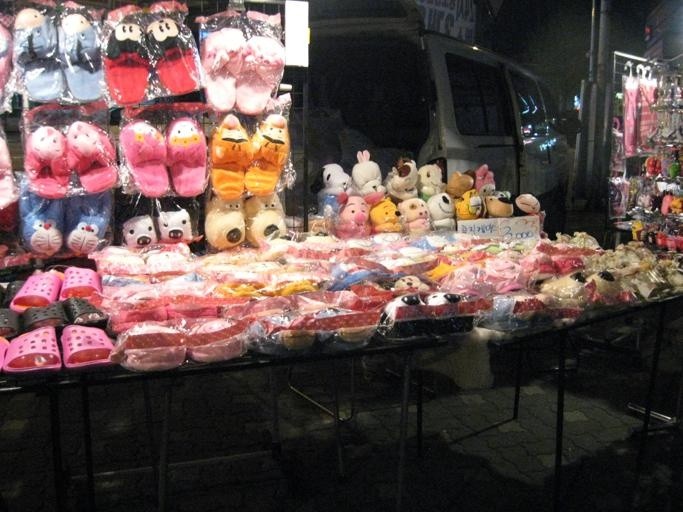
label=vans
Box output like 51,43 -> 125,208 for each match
308,0 -> 582,245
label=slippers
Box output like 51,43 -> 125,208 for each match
0,1 -> 285,118
311,151 -> 541,234
94,234 -> 392,368
1,264 -> 116,377
18,171 -> 288,256
25,113 -> 289,201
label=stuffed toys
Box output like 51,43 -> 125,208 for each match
303,147 -> 551,240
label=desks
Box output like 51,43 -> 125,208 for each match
2,291 -> 682,511
